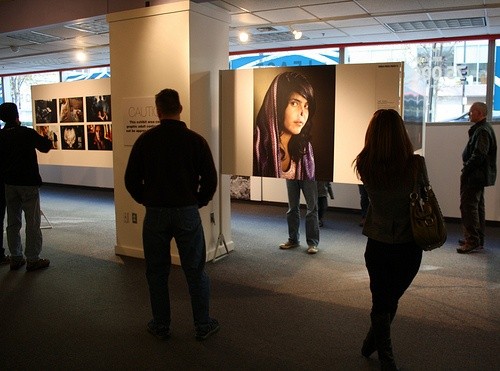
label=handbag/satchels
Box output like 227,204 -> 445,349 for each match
410,153 -> 447,252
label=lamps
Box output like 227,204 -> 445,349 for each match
287,25 -> 304,40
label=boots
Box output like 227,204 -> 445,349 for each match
369,310 -> 400,370
361,305 -> 398,357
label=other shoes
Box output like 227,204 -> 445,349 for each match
0,254 -> 10,264
279,242 -> 299,249
9,257 -> 24,270
456,241 -> 480,253
26,259 -> 50,272
307,245 -> 318,253
458,239 -> 485,249
193,318 -> 219,340
359,217 -> 366,226
147,319 -> 171,340
319,220 -> 324,226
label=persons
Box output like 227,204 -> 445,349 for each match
254,72 -> 317,179
0,102 -> 53,273
0,183 -> 11,264
89,124 -> 111,148
124,88 -> 220,342
456,102 -> 497,254
352,109 -> 431,371
279,179 -> 320,254
315,181 -> 335,228
358,185 -> 369,228
